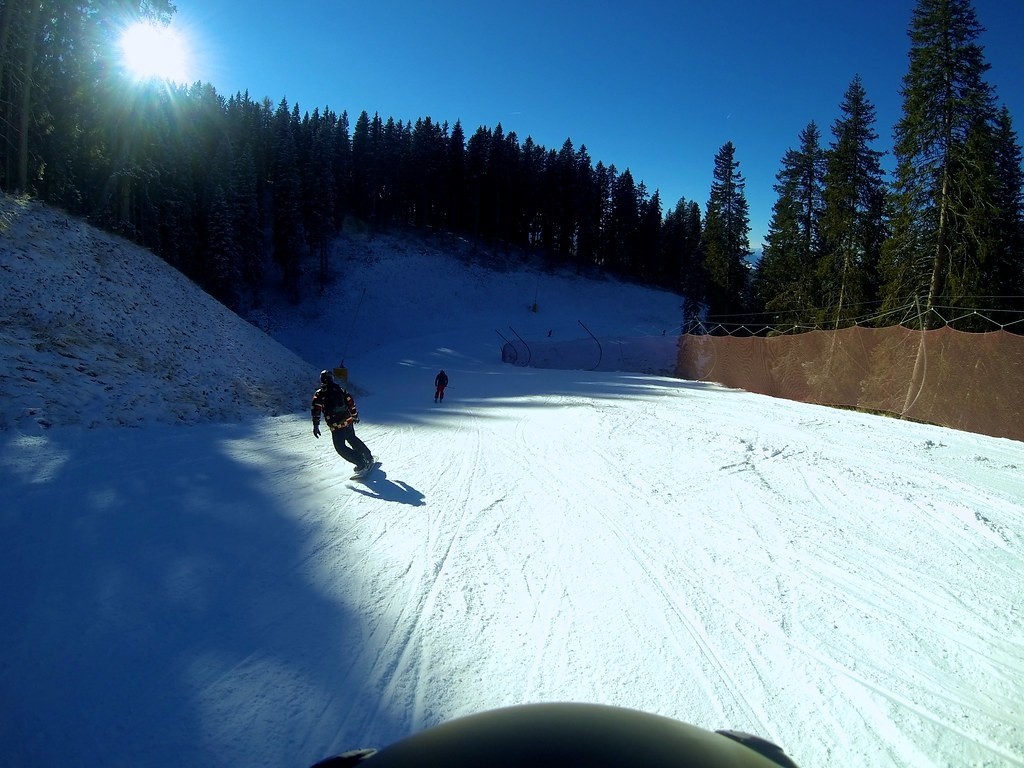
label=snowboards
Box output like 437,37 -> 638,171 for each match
348,455 -> 380,481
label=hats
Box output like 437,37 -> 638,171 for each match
320,370 -> 334,384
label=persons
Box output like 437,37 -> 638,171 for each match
434,370 -> 449,401
311,370 -> 374,470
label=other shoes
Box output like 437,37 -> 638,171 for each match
353,463 -> 367,473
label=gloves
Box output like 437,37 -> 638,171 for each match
353,413 -> 360,424
313,426 -> 321,438
